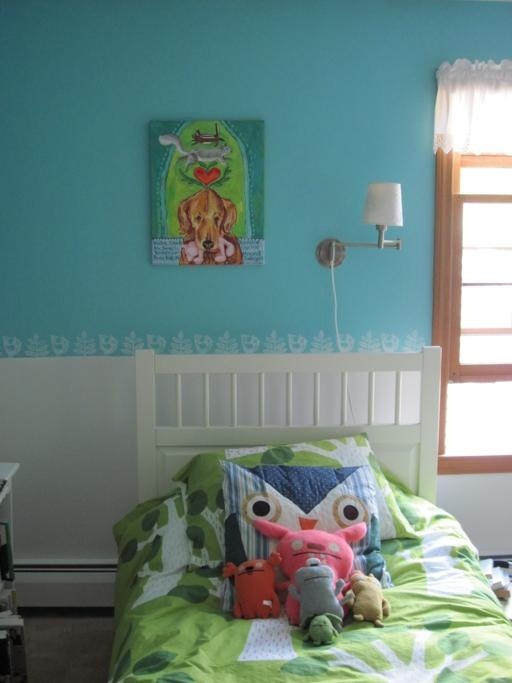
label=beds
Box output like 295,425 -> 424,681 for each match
135,344 -> 509,681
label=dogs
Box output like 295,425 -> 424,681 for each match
177,187 -> 238,254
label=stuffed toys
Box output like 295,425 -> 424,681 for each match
252,517 -> 368,625
221,550 -> 284,619
287,565 -> 347,627
300,612 -> 343,646
345,568 -> 390,627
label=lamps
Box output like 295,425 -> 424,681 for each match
316,180 -> 405,273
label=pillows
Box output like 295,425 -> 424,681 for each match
213,431 -> 426,615
